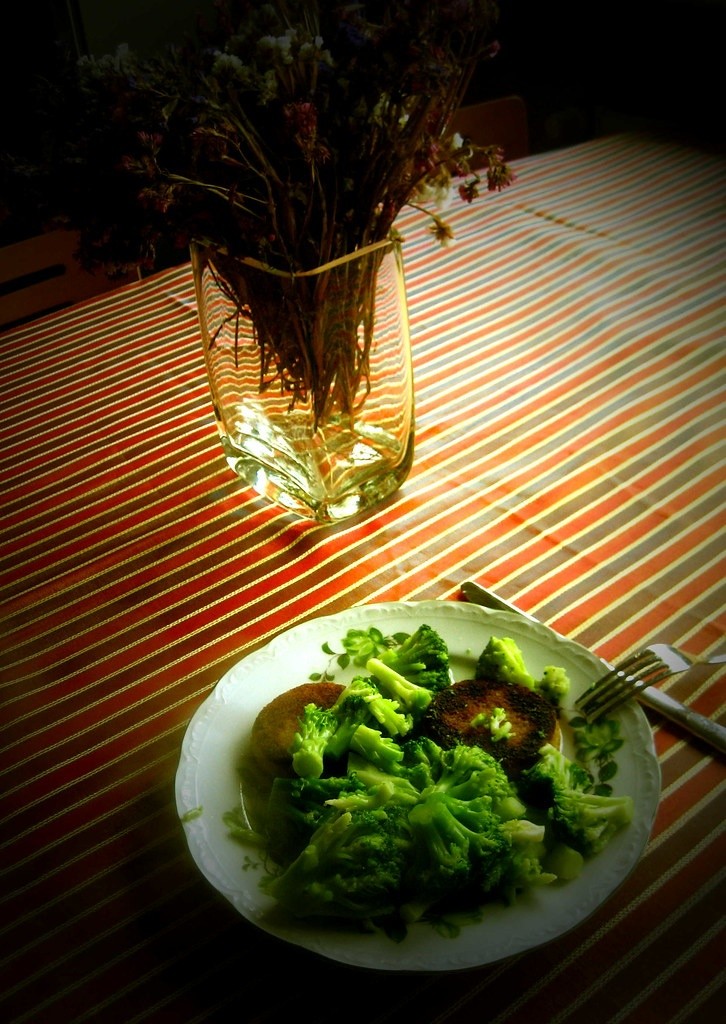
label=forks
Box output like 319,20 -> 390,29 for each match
574,644 -> 726,725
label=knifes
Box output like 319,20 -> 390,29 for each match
462,582 -> 726,759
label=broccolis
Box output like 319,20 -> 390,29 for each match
258,624 -> 633,933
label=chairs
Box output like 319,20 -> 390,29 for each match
0,200 -> 142,325
373,96 -> 529,177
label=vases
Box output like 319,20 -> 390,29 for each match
190,230 -> 414,524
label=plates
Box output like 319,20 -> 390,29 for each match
174,599 -> 663,971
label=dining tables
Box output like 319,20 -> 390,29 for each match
0,127 -> 726,1024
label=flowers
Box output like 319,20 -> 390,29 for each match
0,0 -> 518,432
